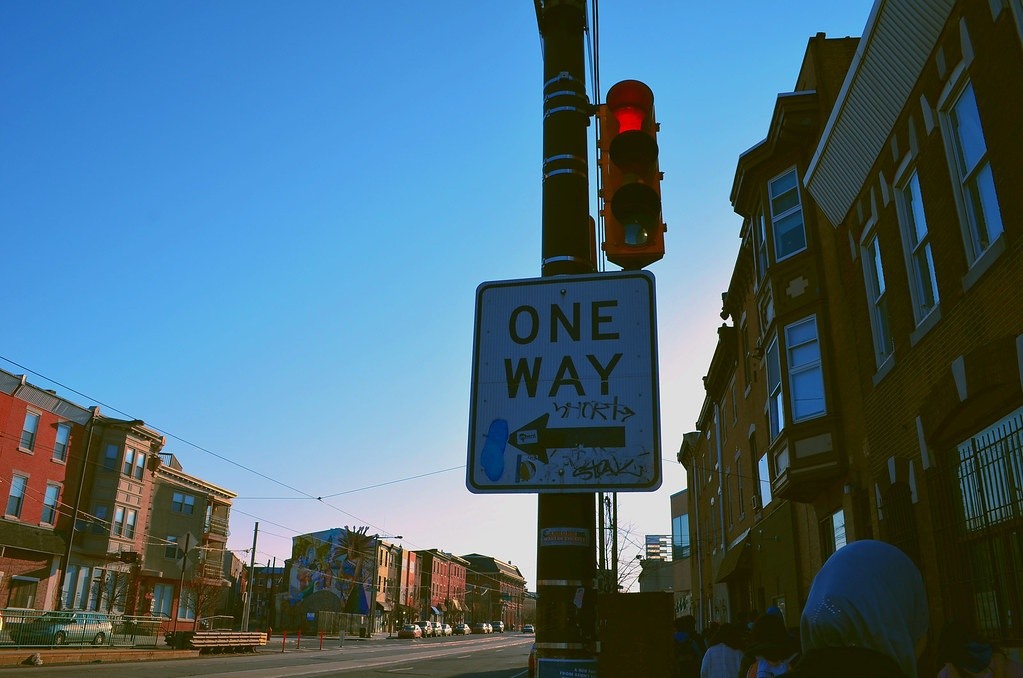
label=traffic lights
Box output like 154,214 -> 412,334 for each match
600,79 -> 665,269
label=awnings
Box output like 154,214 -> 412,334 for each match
376,601 -> 391,611
452,600 -> 469,612
715,539 -> 751,584
438,604 -> 447,611
431,607 -> 439,615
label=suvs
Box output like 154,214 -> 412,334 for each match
431,621 -> 443,637
9,609 -> 114,648
414,620 -> 433,638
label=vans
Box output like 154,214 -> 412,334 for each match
491,621 -> 505,633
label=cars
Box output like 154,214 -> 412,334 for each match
442,624 -> 452,637
397,624 -> 423,639
475,623 -> 492,634
523,624 -> 534,633
453,624 -> 471,636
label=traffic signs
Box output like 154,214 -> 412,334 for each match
465,271 -> 664,493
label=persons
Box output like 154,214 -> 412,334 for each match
674,539 -> 1023,678
